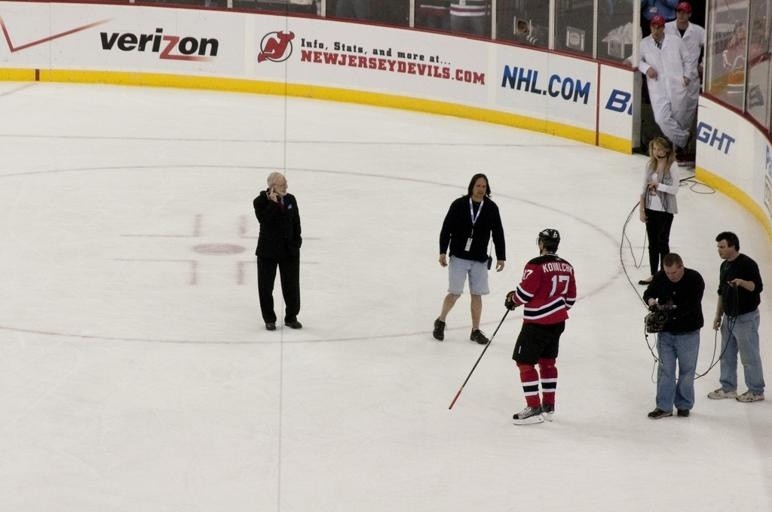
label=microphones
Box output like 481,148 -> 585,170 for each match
658,153 -> 668,158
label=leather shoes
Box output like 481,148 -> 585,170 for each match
286,317 -> 301,329
266,322 -> 274,329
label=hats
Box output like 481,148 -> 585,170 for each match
650,15 -> 664,25
674,2 -> 692,13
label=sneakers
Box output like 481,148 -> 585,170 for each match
433,317 -> 445,340
513,407 -> 545,419
543,406 -> 555,414
469,327 -> 488,345
740,389 -> 764,403
677,408 -> 689,418
709,387 -> 736,398
675,152 -> 693,166
649,408 -> 671,418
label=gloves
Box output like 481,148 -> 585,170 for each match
505,291 -> 513,311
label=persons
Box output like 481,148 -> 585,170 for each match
638,15 -> 694,160
707,232 -> 766,404
639,136 -> 680,284
505,227 -> 576,419
663,2 -> 707,98
642,253 -> 705,418
253,171 -> 302,330
433,173 -> 505,345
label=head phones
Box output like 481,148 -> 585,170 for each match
660,135 -> 673,155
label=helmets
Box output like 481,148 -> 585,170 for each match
537,229 -> 559,248
